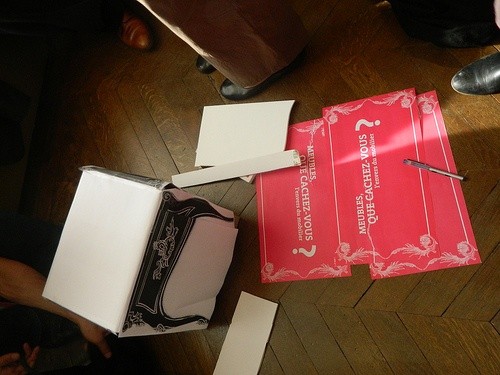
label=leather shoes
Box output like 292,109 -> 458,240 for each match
220,50 -> 308,99
108,5 -> 155,51
450,50 -> 500,96
196,55 -> 216,74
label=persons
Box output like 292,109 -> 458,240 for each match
137,0 -> 308,101
449,0 -> 500,96
0,2 -> 161,373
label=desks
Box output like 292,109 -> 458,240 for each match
138,0 -> 340,89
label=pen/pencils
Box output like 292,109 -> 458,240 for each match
403,158 -> 464,180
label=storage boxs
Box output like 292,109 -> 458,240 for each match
42,166 -> 240,339
